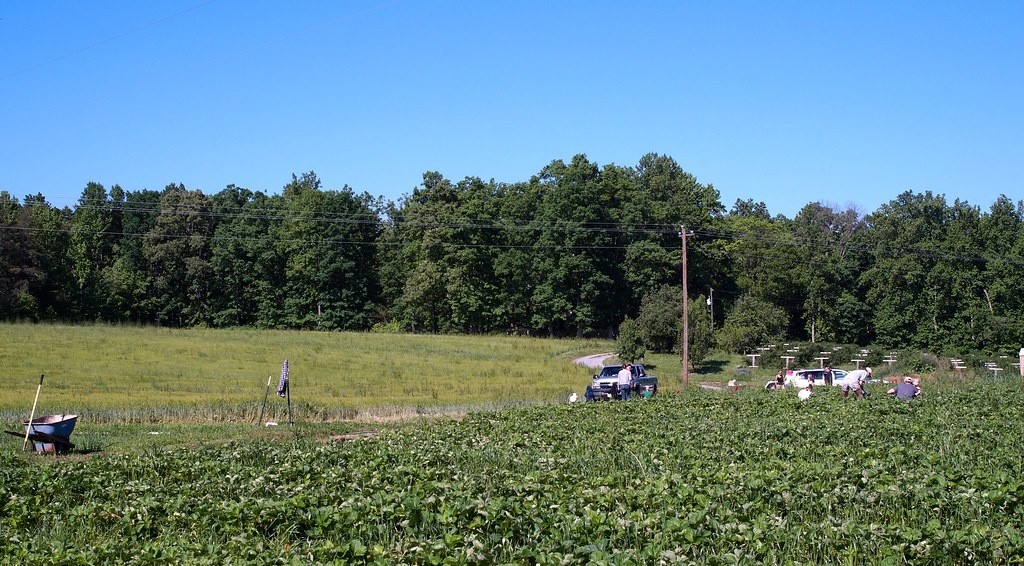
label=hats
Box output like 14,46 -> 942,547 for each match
866,367 -> 873,378
912,379 -> 920,386
904,377 -> 912,382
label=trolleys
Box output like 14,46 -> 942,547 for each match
3,412 -> 80,456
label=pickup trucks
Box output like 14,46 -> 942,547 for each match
586,364 -> 658,405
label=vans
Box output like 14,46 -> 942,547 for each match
764,369 -> 850,393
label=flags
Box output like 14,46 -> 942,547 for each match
276,359 -> 290,398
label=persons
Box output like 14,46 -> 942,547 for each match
617,362 -> 633,402
887,377 -> 921,401
775,370 -> 787,390
805,373 -> 814,392
1019,348 -> 1024,377
727,378 -> 735,387
822,366 -> 832,387
842,367 -> 872,399
